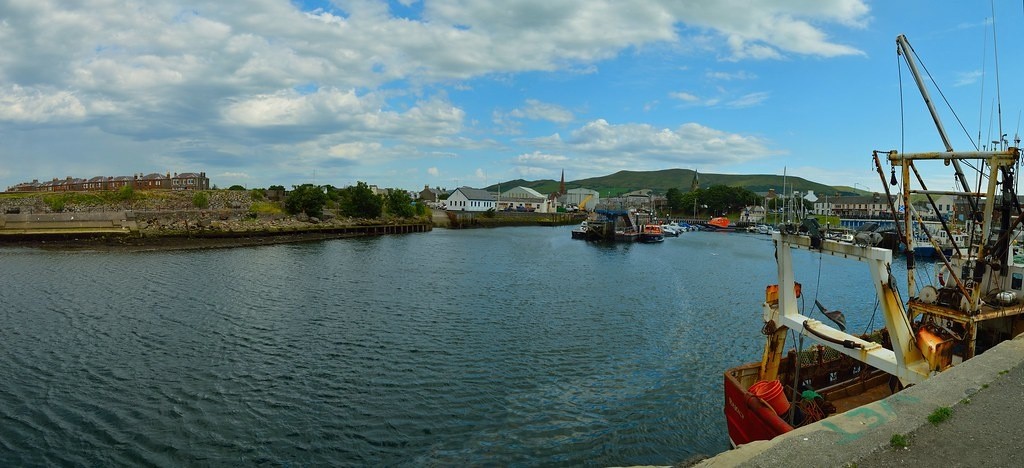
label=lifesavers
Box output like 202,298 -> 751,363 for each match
938,265 -> 947,287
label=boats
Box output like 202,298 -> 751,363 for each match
571,0 -> 1024,451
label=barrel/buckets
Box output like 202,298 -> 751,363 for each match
748,380 -> 791,416
